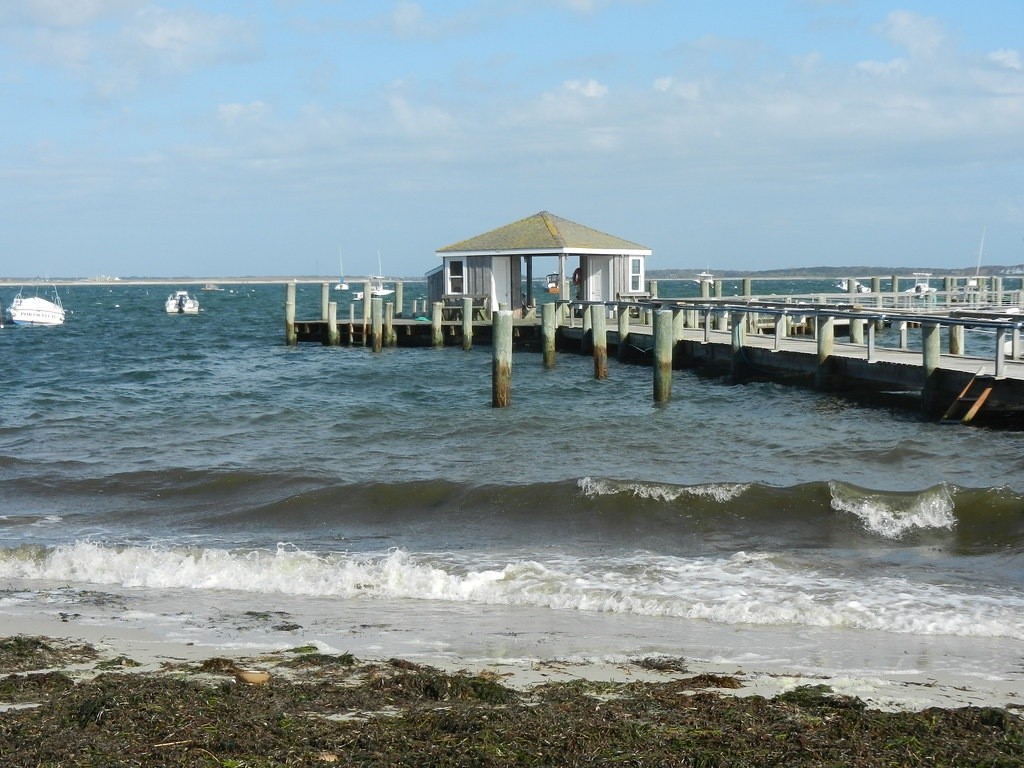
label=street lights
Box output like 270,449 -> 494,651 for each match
904,272 -> 937,300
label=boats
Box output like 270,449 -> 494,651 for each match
165,290 -> 201,314
943,275 -> 989,302
335,283 -> 349,290
834,277 -> 872,293
352,274 -> 396,301
201,283 -> 225,290
541,271 -> 563,294
5,283 -> 65,328
